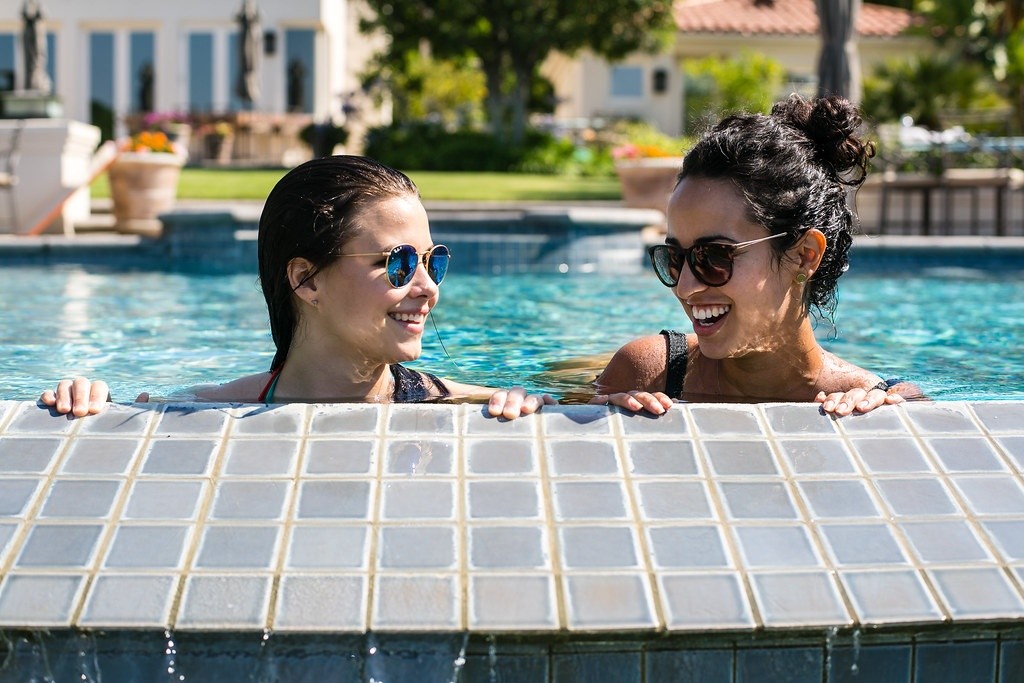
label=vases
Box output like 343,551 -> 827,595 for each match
107,153 -> 186,236
614,158 -> 682,216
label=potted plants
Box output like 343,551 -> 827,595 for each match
300,122 -> 351,159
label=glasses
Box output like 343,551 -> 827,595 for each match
647,232 -> 788,288
335,244 -> 450,289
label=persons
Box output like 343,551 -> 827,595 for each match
585,90 -> 925,417
39,154 -> 561,420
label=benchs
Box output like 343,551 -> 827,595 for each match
874,167 -> 1024,237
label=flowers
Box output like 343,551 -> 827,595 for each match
609,140 -> 683,160
117,127 -> 178,153
